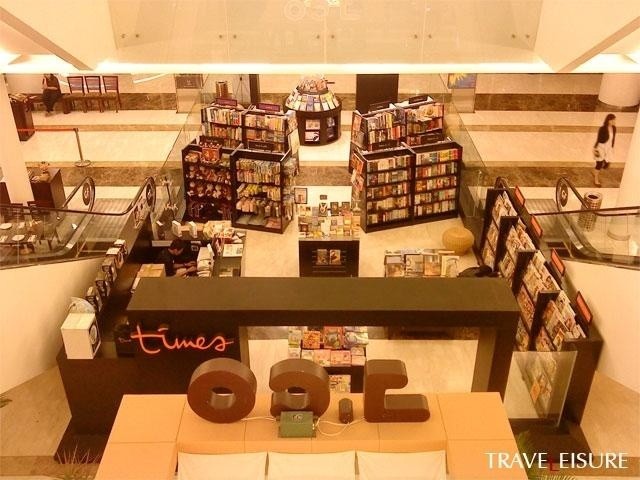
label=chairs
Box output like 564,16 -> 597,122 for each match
66,75 -> 122,113
4,200 -> 60,250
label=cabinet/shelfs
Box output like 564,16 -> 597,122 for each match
348,92 -> 462,234
298,215 -> 360,277
180,97 -> 300,235
478,184 -> 604,426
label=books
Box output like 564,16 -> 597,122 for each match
383,191 -> 588,416
288,324 -> 369,394
203,90 -> 341,154
350,102 -> 458,227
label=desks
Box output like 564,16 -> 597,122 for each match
283,323 -> 368,391
0,221 -> 45,253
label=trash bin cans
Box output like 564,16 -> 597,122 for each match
216,81 -> 227,100
578,191 -> 602,232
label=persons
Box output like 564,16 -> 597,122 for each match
592,114 -> 618,187
154,239 -> 198,278
41,73 -> 61,116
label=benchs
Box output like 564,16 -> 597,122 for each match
26,93 -> 70,115
